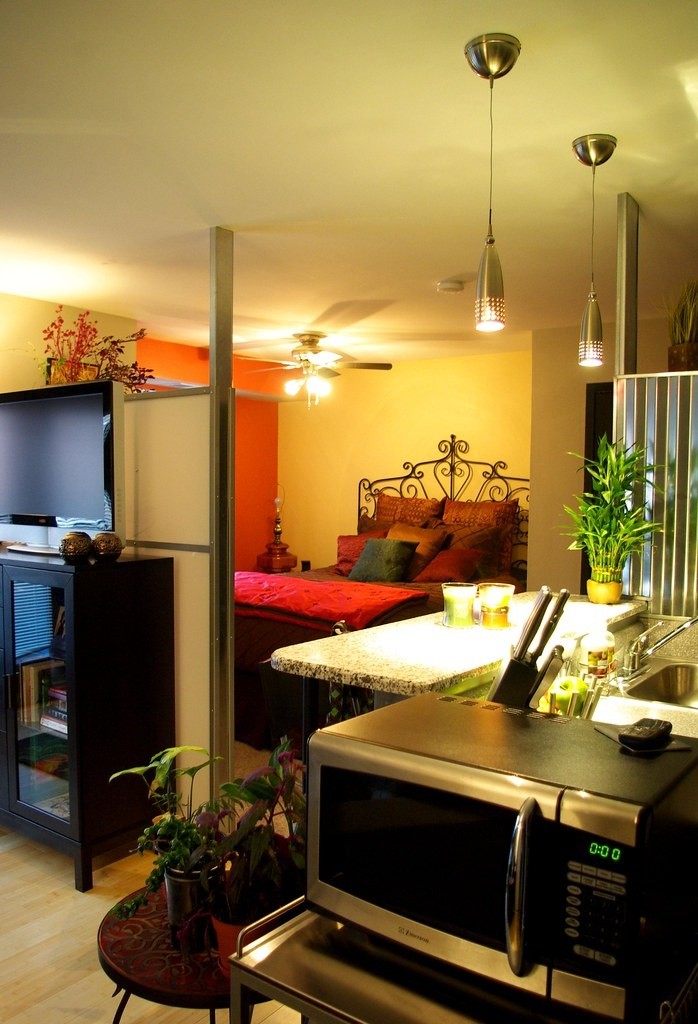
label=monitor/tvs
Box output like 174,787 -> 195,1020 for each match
0,380 -> 124,557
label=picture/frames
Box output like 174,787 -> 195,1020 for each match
45,356 -> 101,383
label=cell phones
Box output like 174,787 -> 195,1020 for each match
618,718 -> 673,747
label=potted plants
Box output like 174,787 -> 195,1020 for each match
660,275 -> 698,372
549,435 -> 670,608
103,736 -> 317,977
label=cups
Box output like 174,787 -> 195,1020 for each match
441,582 -> 478,627
478,582 -> 515,627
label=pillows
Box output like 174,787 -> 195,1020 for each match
415,545 -> 489,582
441,496 -> 521,573
347,536 -> 420,583
333,532 -> 383,574
429,519 -> 502,579
375,492 -> 441,523
385,521 -> 449,580
356,513 -> 426,534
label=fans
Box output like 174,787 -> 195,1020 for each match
233,334 -> 394,378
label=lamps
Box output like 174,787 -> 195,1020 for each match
256,481 -> 297,573
280,365 -> 338,407
572,132 -> 618,373
460,30 -> 521,335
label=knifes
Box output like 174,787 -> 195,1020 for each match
512,582 -> 570,711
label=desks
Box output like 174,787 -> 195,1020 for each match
92,883 -> 272,1024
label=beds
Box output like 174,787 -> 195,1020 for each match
232,432 -> 535,758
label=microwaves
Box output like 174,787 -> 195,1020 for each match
304,694 -> 697,1024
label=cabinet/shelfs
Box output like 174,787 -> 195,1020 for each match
0,541 -> 176,895
